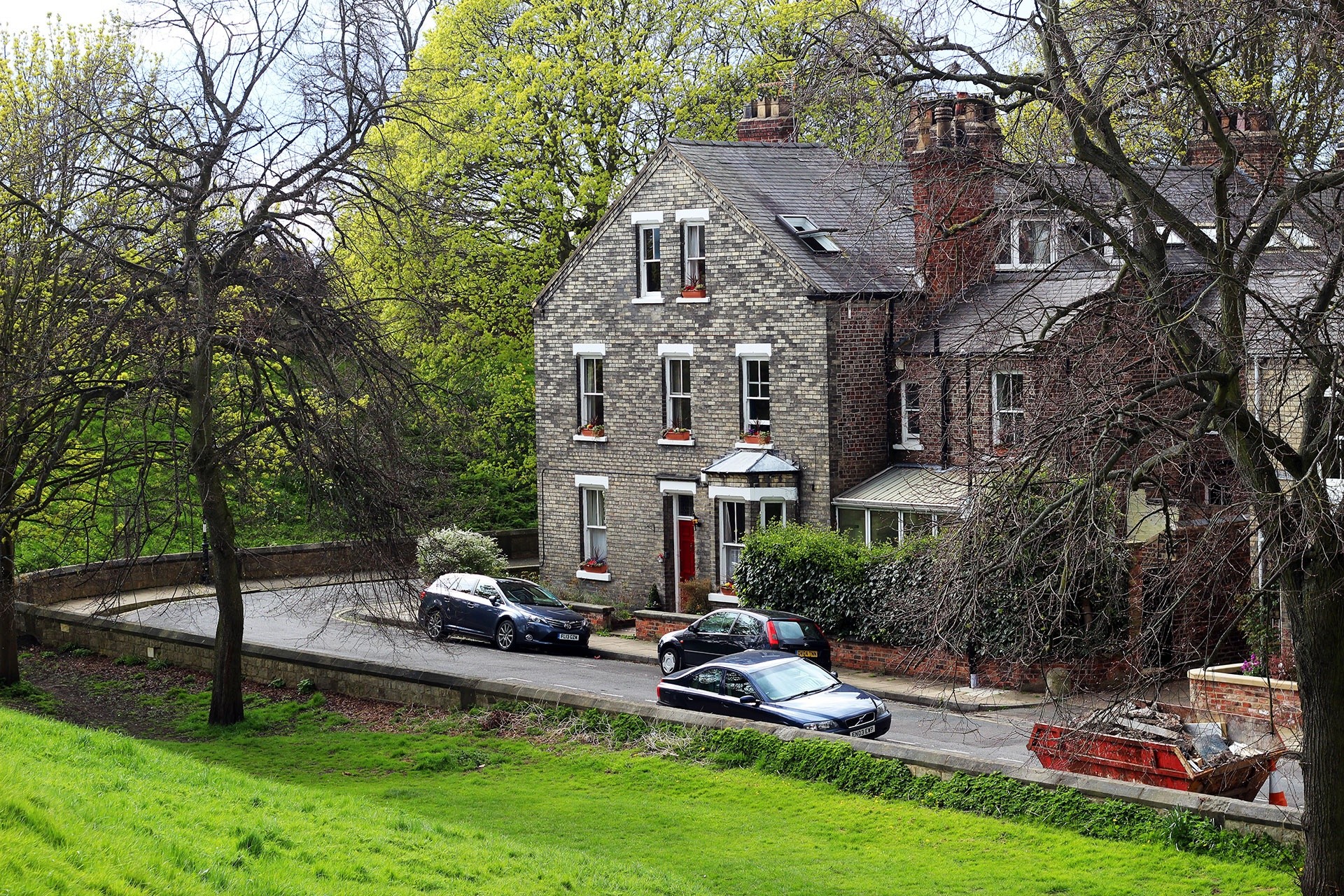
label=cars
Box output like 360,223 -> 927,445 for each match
656,650 -> 892,740
418,573 -> 591,651
658,608 -> 838,677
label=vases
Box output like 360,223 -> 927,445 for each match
721,585 -> 739,595
746,434 -> 769,445
682,287 -> 704,299
666,431 -> 691,441
583,564 -> 607,573
581,428 -> 604,437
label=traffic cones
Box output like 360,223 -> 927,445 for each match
1269,758 -> 1288,807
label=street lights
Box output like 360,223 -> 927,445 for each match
960,499 -> 978,688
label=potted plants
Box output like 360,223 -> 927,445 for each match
644,583 -> 664,611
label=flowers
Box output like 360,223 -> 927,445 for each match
579,548 -> 608,566
577,418 -> 608,438
721,578 -> 742,589
679,274 -> 710,292
661,419 -> 695,437
740,424 -> 777,438
